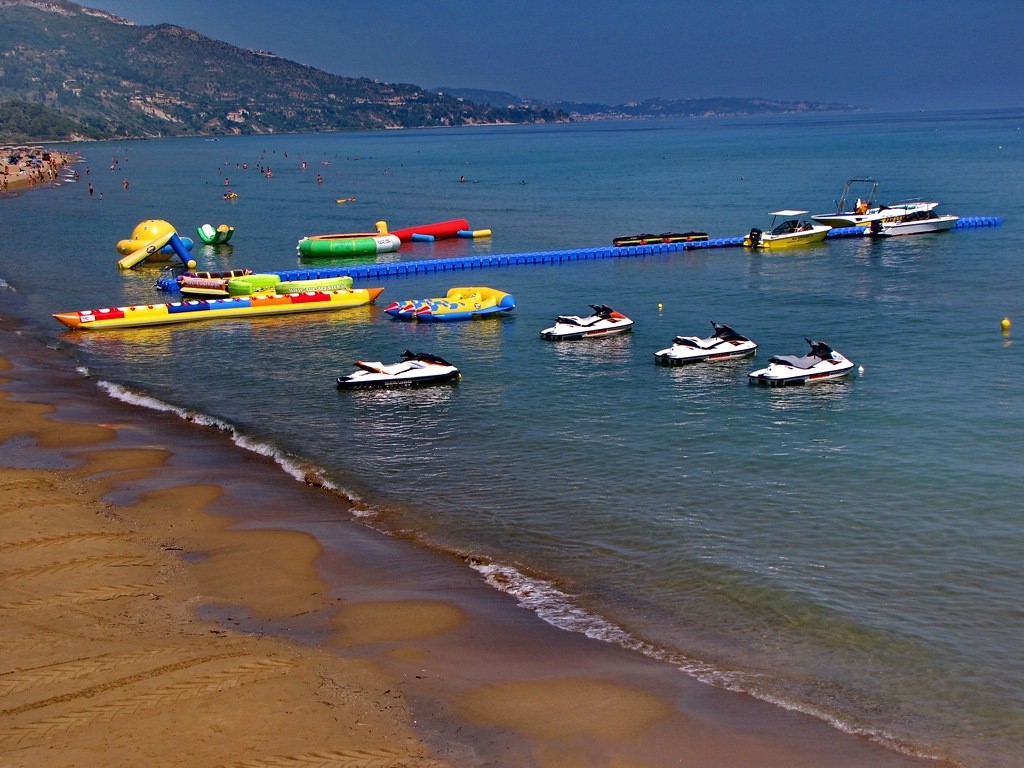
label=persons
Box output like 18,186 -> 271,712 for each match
853,198 -> 872,215
0,145 -> 465,201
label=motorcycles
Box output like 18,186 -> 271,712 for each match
539,304 -> 635,340
654,321 -> 758,365
336,350 -> 462,390
747,338 -> 855,387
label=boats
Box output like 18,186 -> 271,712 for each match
52,269 -> 384,330
296,221 -> 401,257
743,210 -> 832,248
375,219 -> 491,242
612,231 -> 709,247
197,224 -> 234,244
811,176 -> 960,236
384,286 -> 516,321
